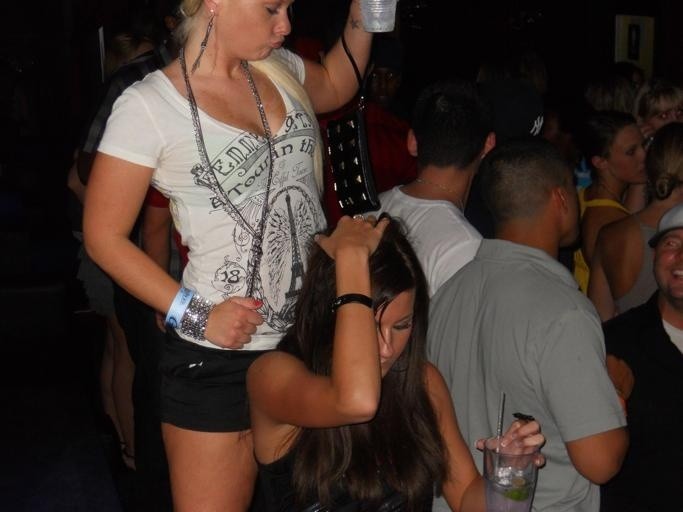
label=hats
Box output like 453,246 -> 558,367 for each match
648,201 -> 683,248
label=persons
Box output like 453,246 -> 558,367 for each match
294,32 -> 681,319
245,212 -> 546,511
66,0 -> 193,476
601,202 -> 682,511
83,1 -> 374,511
426,135 -> 635,511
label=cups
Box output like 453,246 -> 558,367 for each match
359,0 -> 399,33
484,435 -> 539,512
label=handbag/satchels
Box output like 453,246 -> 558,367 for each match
326,111 -> 381,216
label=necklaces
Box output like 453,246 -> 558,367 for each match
179,45 -> 276,299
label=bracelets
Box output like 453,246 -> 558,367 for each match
179,294 -> 216,341
